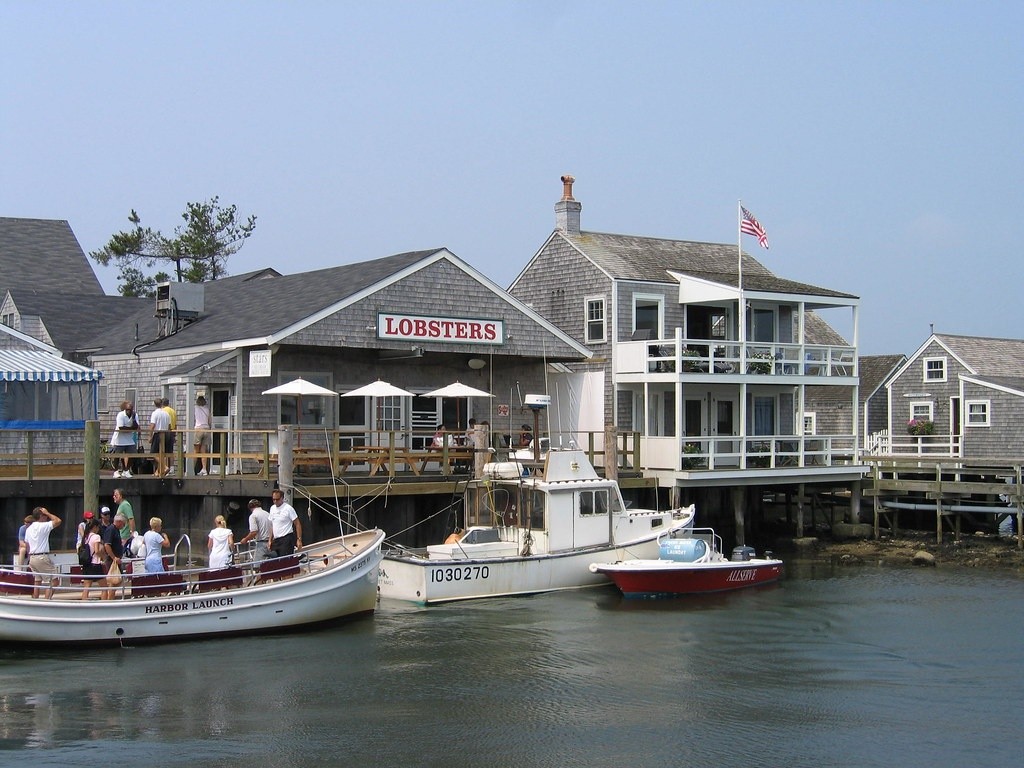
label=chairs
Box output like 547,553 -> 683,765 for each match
131,573 -> 186,601
0,574 -> 35,596
199,567 -> 244,594
658,347 -> 732,373
70,565 -> 108,583
261,557 -> 300,584
125,557 -> 170,580
805,440 -> 818,465
817,351 -> 847,377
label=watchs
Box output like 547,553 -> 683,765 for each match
297,537 -> 302,540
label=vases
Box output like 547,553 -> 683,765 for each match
682,364 -> 689,371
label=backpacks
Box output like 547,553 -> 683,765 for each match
78,534 -> 95,565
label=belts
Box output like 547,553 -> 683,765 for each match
257,540 -> 268,542
30,553 -> 48,555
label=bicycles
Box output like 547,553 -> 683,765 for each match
99,439 -> 125,472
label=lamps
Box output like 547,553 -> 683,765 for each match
746,300 -> 752,310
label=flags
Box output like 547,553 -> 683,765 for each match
741,205 -> 769,249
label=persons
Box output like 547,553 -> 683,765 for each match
241,499 -> 274,568
18,507 -> 62,600
519,424 -> 534,446
76,487 -> 170,600
194,396 -> 212,476
432,418 -> 493,475
268,489 -> 302,557
148,397 -> 177,477
208,515 -> 234,570
110,400 -> 142,479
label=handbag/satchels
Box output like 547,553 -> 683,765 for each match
106,559 -> 123,584
137,543 -> 146,558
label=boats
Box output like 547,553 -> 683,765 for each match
588,527 -> 785,601
376,337 -> 696,604
0,426 -> 386,650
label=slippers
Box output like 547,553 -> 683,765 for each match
153,469 -> 170,477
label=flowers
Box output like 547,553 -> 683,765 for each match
907,416 -> 934,436
664,348 -> 703,370
749,350 -> 783,373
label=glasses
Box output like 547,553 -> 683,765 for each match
102,513 -> 110,516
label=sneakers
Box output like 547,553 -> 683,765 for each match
122,470 -> 132,478
113,470 -> 121,478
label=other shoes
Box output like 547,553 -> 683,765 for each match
197,469 -> 207,476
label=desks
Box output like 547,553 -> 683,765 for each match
777,440 -> 798,466
420,446 -> 474,475
258,447 -> 330,477
340,446 -> 420,478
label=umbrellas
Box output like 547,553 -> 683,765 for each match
261,377 -> 338,475
419,380 -> 497,467
341,379 -> 416,475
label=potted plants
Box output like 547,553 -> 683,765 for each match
682,444 -> 707,470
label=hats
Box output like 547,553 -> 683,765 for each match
84,511 -> 95,518
101,507 -> 111,515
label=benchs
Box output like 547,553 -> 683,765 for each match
260,457 -> 473,463
